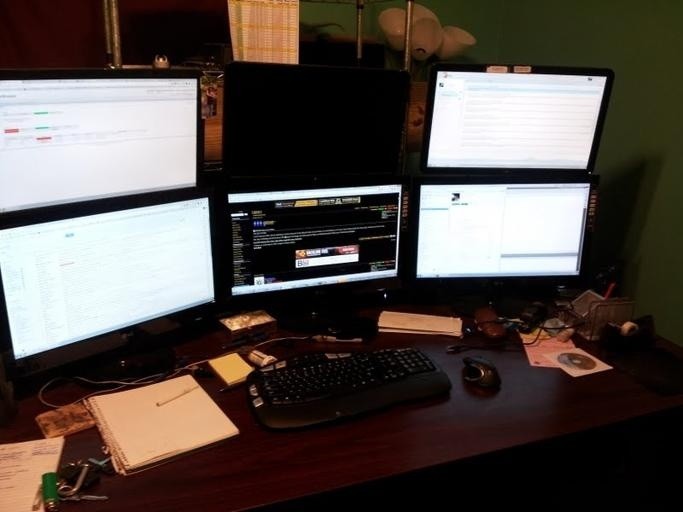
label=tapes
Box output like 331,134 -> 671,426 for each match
620,321 -> 640,336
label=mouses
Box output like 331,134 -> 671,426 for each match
462,356 -> 504,391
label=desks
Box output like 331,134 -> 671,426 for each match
0,302 -> 683,512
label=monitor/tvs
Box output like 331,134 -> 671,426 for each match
0,195 -> 220,376
423,66 -> 613,180
220,185 -> 404,304
414,185 -> 599,296
1,70 -> 206,221
218,62 -> 409,186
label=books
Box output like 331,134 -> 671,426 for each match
85,374 -> 242,475
0,434 -> 67,512
217,309 -> 276,337
376,308 -> 464,339
34,401 -> 97,442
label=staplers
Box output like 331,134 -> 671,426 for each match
518,301 -> 549,334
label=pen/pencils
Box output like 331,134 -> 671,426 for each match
219,381 -> 246,391
157,386 -> 198,407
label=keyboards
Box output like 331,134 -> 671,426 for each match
243,344 -> 455,432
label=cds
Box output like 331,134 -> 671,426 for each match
557,351 -> 598,372
